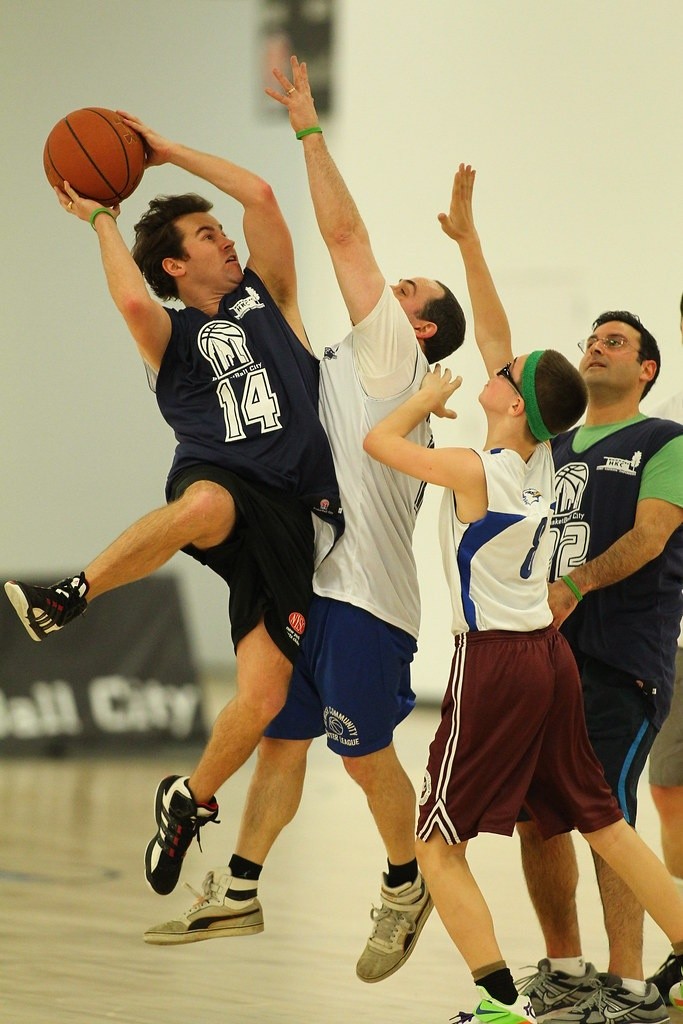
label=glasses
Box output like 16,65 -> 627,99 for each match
496,362 -> 523,398
578,337 -> 650,361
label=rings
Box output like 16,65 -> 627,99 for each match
67,201 -> 73,210
286,88 -> 296,96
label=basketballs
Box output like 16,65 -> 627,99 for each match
42,106 -> 147,208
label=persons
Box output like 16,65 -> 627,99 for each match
3,110 -> 347,893
363,162 -> 683,1022
143,53 -> 466,986
516,309 -> 682,1024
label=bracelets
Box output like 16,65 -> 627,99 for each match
562,575 -> 583,601
296,127 -> 323,141
90,208 -> 117,233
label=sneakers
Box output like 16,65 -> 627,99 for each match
143,866 -> 264,945
4,572 -> 90,641
543,972 -> 671,1024
645,954 -> 683,1012
356,872 -> 434,984
145,775 -> 219,895
514,958 -> 598,1024
450,986 -> 537,1024
